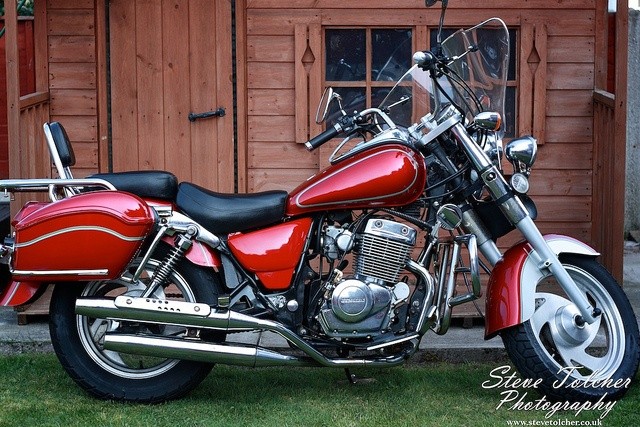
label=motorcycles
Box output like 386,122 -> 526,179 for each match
1,0 -> 639,405
